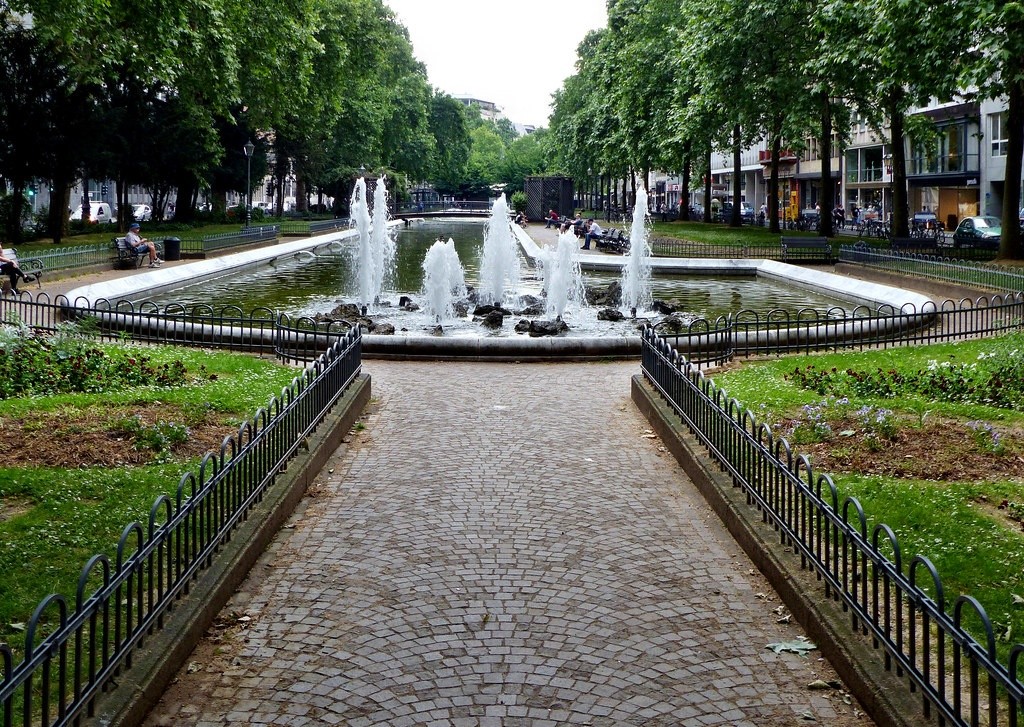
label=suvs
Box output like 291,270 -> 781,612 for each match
68,201 -> 113,223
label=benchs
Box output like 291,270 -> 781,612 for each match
936,235 -> 976,260
112,237 -> 162,267
0,248 -> 44,291
780,235 -> 832,265
595,228 -> 625,252
556,216 -> 590,238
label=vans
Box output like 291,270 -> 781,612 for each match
723,202 -> 755,222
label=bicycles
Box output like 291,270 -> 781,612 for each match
857,218 -> 892,241
912,220 -> 946,245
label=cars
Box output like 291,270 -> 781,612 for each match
952,216 -> 1002,249
131,204 -> 153,221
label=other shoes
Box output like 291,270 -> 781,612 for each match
12,289 -> 21,295
23,276 -> 35,283
148,263 -> 159,268
851,229 -> 853,231
154,258 -> 165,263
580,247 -> 589,250
546,226 -> 550,228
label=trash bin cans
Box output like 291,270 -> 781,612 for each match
163,237 -> 181,261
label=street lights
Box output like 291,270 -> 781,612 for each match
242,137 -> 255,228
779,155 -> 788,229
725,171 -> 732,210
691,171 -> 696,208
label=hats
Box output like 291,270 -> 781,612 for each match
129,223 -> 140,229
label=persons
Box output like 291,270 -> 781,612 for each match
660,201 -> 669,222
544,209 -> 558,228
124,223 -> 165,268
815,202 -> 882,231
0,244 -> 35,296
580,219 -> 603,250
760,203 -> 768,220
556,214 -> 580,235
515,211 -> 528,229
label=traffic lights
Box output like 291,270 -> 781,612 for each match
266,183 -> 274,196
28,177 -> 34,195
35,183 -> 39,194
102,185 -> 108,195
